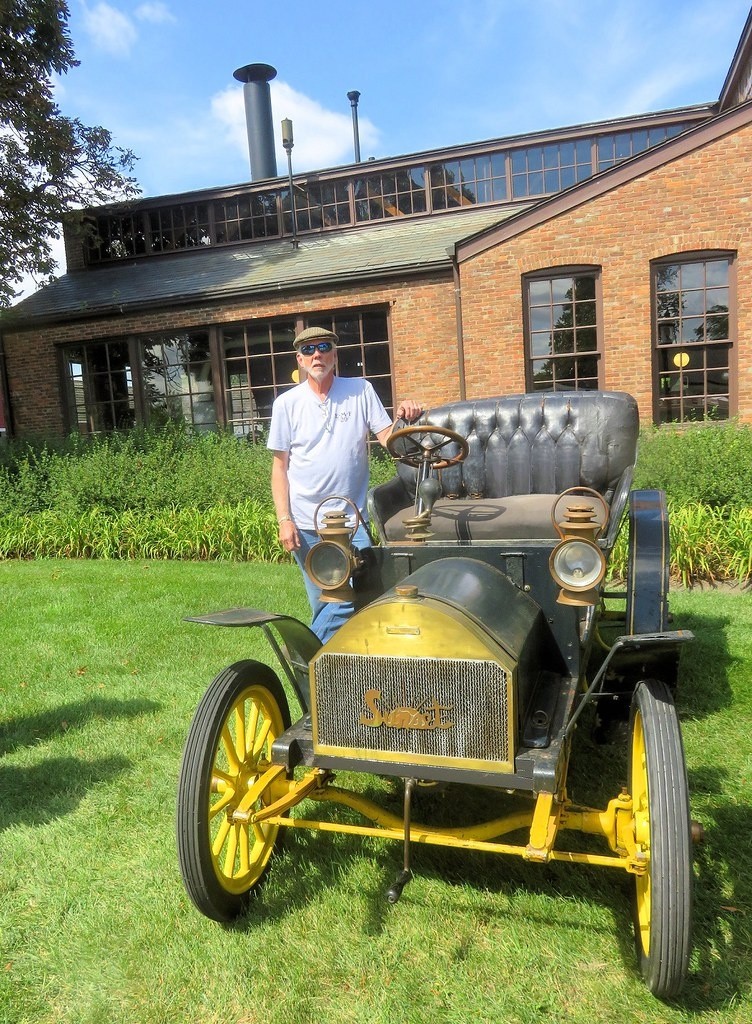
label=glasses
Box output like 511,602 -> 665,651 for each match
301,342 -> 332,355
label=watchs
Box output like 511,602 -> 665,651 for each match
278,515 -> 292,523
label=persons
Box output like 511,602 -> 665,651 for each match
265,326 -> 424,645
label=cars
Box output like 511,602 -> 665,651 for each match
175,390 -> 694,998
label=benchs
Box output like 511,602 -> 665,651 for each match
368,391 -> 639,549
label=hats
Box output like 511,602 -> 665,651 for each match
293,327 -> 339,351
